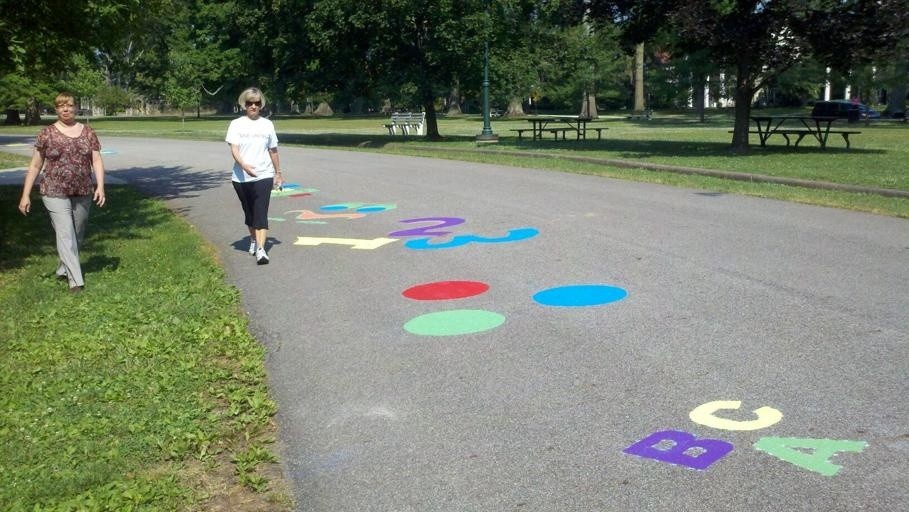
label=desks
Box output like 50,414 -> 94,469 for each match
519,115 -> 592,141
750,115 -> 838,148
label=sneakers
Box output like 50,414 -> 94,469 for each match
248,238 -> 256,256
54,272 -> 86,294
255,247 -> 270,266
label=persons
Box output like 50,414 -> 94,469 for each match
226,86 -> 285,264
18,93 -> 106,293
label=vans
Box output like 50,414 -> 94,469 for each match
810,100 -> 880,119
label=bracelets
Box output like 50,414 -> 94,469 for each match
275,171 -> 282,174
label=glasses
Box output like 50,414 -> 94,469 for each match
245,101 -> 262,106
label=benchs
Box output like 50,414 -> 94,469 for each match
771,130 -> 861,148
510,129 -> 575,140
381,112 -> 425,136
624,111 -> 652,121
550,128 -> 608,141
727,130 -> 817,147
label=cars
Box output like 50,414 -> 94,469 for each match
490,110 -> 504,118
